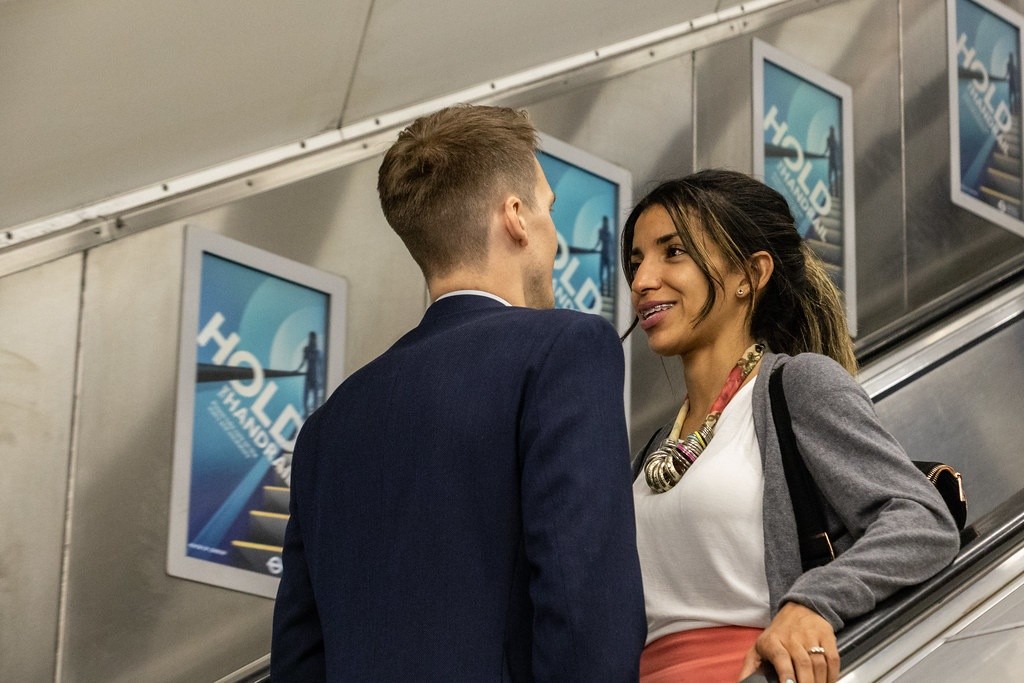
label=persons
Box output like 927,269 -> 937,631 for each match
268,104 -> 644,683
621,168 -> 961,683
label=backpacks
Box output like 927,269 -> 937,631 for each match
770,354 -> 967,584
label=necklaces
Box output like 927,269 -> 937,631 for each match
641,342 -> 764,491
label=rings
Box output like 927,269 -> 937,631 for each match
807,646 -> 826,656
